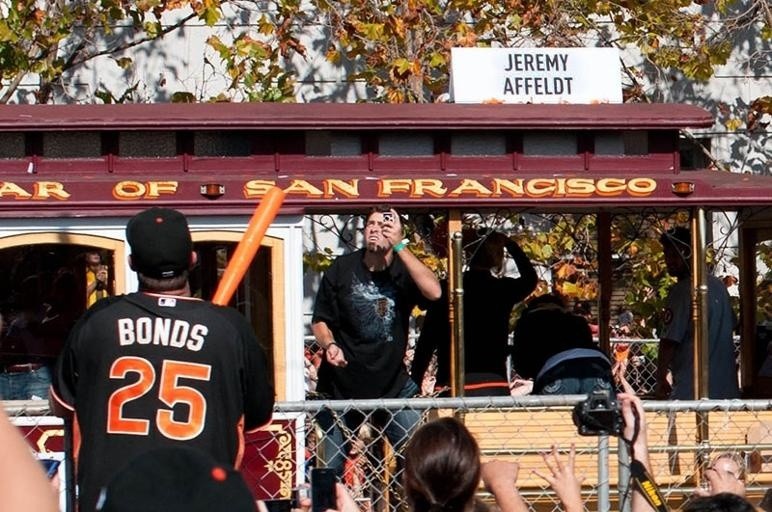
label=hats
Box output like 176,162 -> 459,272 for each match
125,208 -> 192,279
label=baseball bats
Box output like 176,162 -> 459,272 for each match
212,187 -> 285,306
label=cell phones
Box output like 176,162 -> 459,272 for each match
311,468 -> 338,512
38,459 -> 61,482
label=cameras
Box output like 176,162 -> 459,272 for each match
572,387 -> 626,436
382,212 -> 394,222
